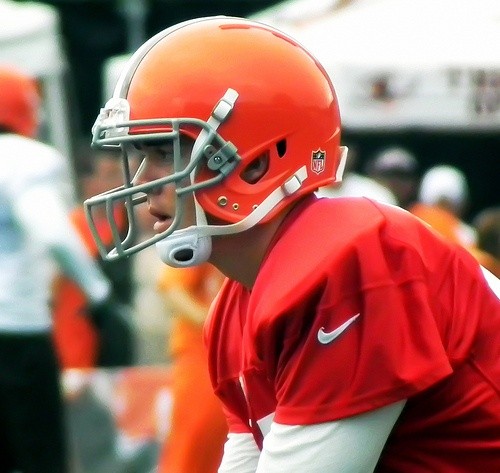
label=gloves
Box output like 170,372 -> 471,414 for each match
88,301 -> 134,368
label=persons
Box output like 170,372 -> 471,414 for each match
54,139 -> 500,371
0,63 -> 154,473
85,16 -> 499,472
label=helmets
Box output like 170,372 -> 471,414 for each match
0,65 -> 40,139
420,165 -> 469,211
82,10 -> 349,270
375,147 -> 421,180
